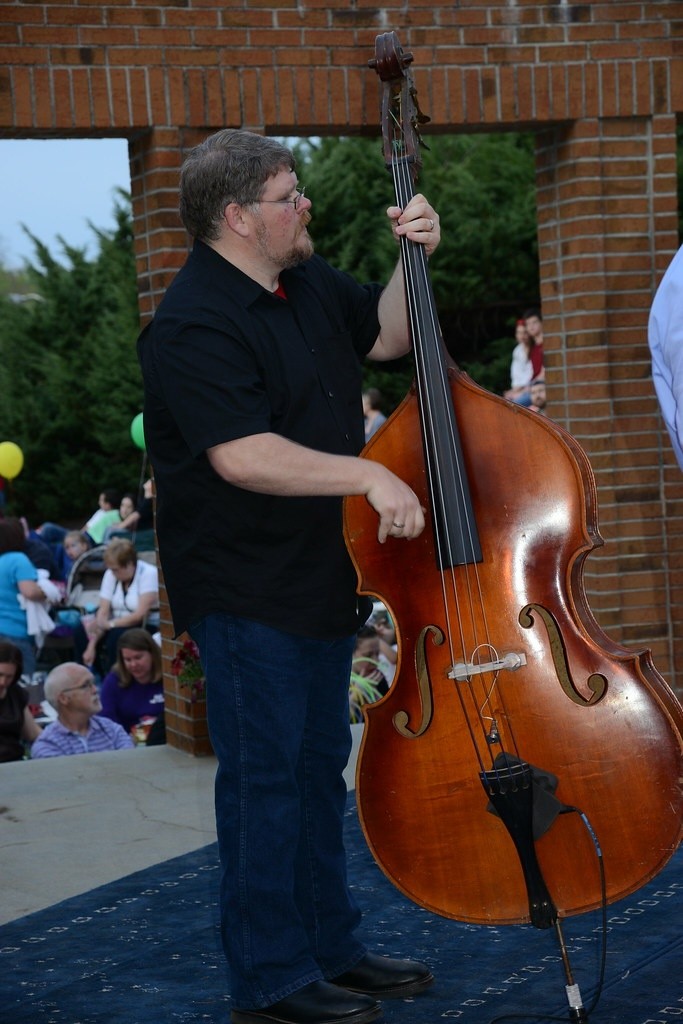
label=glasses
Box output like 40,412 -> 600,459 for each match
249,186 -> 306,210
62,679 -> 96,697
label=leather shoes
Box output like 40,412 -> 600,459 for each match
230,980 -> 384,1024
325,952 -> 435,999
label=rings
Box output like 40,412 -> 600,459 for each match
429,219 -> 434,231
393,520 -> 406,528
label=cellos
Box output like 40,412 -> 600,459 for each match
341,29 -> 682,1023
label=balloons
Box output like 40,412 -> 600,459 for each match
0,441 -> 24,480
130,413 -> 145,449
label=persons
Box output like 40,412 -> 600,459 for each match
347,622 -> 398,725
0,478 -> 162,764
361,386 -> 390,442
503,310 -> 546,419
648,244 -> 683,475
136,129 -> 439,1024
